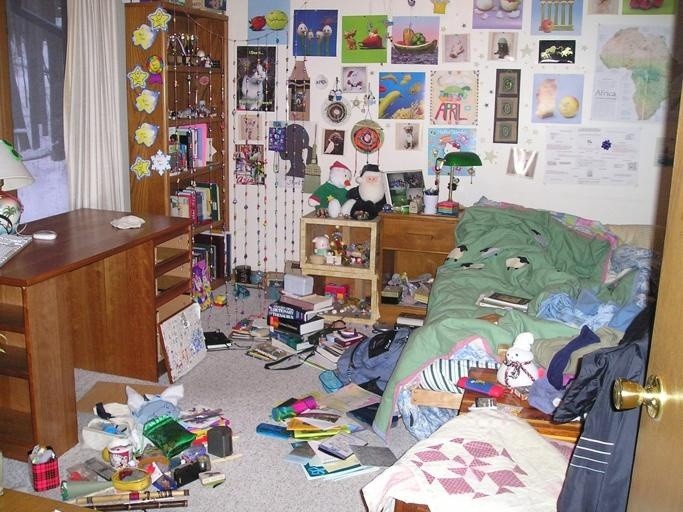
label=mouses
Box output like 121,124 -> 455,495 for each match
33,230 -> 58,240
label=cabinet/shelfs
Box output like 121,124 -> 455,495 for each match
300,269 -> 381,326
125,2 -> 229,304
378,208 -> 461,324
300,210 -> 382,276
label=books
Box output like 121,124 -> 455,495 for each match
191,230 -> 232,285
170,182 -> 220,225
267,291 -> 333,354
476,292 -> 531,311
169,124 -> 207,175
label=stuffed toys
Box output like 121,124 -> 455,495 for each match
346,165 -> 386,219
312,233 -> 349,263
497,332 -> 538,387
308,162 -> 352,218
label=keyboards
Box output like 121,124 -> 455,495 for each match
0,231 -> 32,272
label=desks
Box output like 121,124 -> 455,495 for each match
0,208 -> 196,464
394,366 -> 585,512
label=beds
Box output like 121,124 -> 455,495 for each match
372,195 -> 667,446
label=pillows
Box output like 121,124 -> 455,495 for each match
533,328 -> 625,380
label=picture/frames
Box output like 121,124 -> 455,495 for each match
383,169 -> 426,207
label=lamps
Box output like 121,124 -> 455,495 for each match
437,151 -> 483,216
0,138 -> 35,238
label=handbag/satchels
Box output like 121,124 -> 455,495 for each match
263,319 -> 347,371
